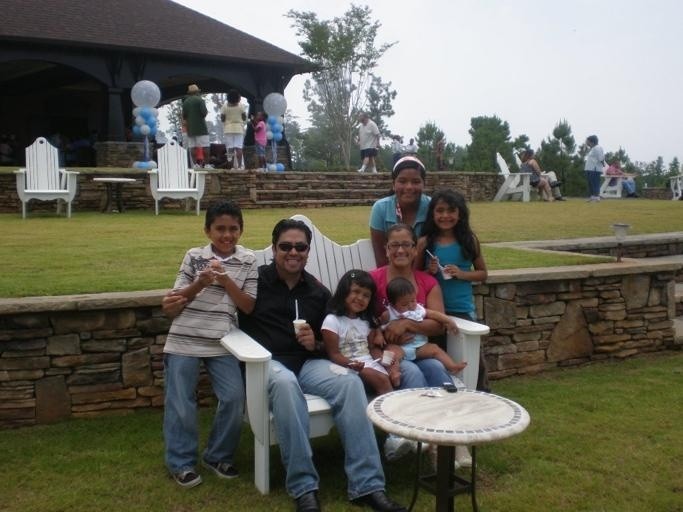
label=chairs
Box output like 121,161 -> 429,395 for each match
493,150 -> 561,202
147,140 -> 208,216
669,175 -> 683,200
13,136 -> 80,219
600,161 -> 624,199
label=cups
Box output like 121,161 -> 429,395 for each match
380,349 -> 395,366
439,264 -> 457,281
292,318 -> 307,335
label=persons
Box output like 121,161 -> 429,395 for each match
583,134 -> 607,203
372,277 -> 468,388
160,216 -> 405,512
412,188 -> 491,393
405,139 -> 417,154
520,149 -> 555,202
181,84 -> 215,172
367,154 -> 434,269
433,134 -> 447,171
319,268 -> 431,465
354,114 -> 380,174
390,134 -> 404,166
249,111 -> 267,172
517,147 -> 567,201
159,198 -> 260,492
369,221 -> 478,472
605,160 -> 640,198
217,88 -> 246,170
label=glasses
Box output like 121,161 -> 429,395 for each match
388,242 -> 415,249
279,243 -> 308,252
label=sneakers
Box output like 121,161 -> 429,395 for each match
357,169 -> 377,174
171,467 -> 203,489
384,436 -> 412,461
551,182 -> 561,188
196,164 -> 269,173
203,455 -> 241,481
586,197 -> 600,203
544,196 -> 566,201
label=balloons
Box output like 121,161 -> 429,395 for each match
130,78 -> 162,170
260,89 -> 288,172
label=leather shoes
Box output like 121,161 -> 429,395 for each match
295,490 -> 321,512
348,489 -> 407,512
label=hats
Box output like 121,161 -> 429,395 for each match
187,84 -> 202,94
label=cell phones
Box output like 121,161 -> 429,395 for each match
442,382 -> 457,392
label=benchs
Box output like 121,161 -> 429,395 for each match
220,213 -> 490,496
